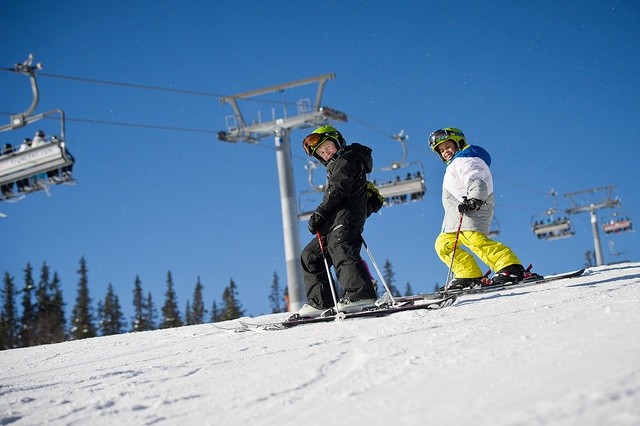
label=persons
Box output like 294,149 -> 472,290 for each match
601,214 -> 635,235
396,176 -> 405,206
426,125 -> 527,293
387,179 -> 394,206
534,214 -> 571,239
1,138 -> 21,202
403,171 -> 416,204
286,122 -> 374,320
14,134 -> 38,199
33,126 -> 78,188
415,170 -> 424,201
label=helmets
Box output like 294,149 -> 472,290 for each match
431,128 -> 465,162
312,125 -> 346,166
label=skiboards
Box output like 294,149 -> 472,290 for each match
280,295 -> 457,329
432,268 -> 585,299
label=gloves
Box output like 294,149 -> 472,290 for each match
458,198 -> 482,212
309,205 -> 329,235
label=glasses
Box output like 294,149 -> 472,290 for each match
302,131 -> 341,156
428,129 -> 465,148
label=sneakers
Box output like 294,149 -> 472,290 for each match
444,278 -> 484,291
490,266 -> 526,283
333,292 -> 377,315
293,302 -> 334,317
